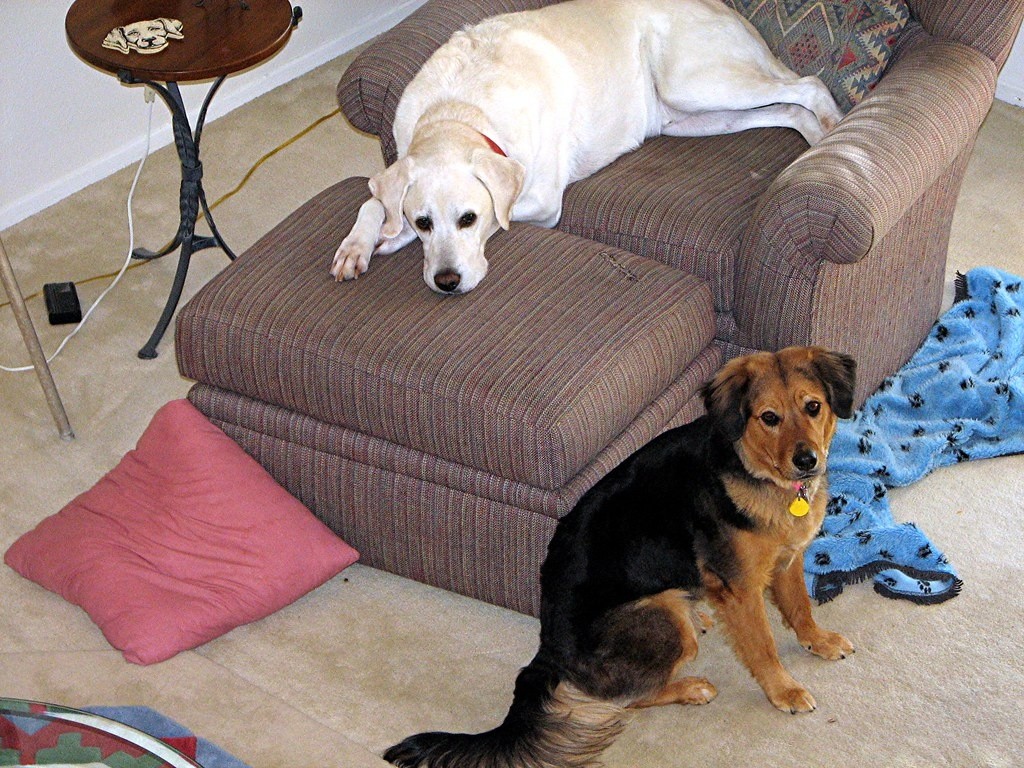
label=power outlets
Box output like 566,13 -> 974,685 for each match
291,1 -> 298,24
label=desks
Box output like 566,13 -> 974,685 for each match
65,0 -> 293,360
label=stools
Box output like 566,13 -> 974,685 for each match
175,177 -> 724,618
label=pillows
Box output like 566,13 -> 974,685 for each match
722,0 -> 909,114
3,401 -> 361,665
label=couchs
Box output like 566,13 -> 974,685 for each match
334,0 -> 1024,408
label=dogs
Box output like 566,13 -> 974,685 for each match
329,1 -> 844,295
383,347 -> 858,768
